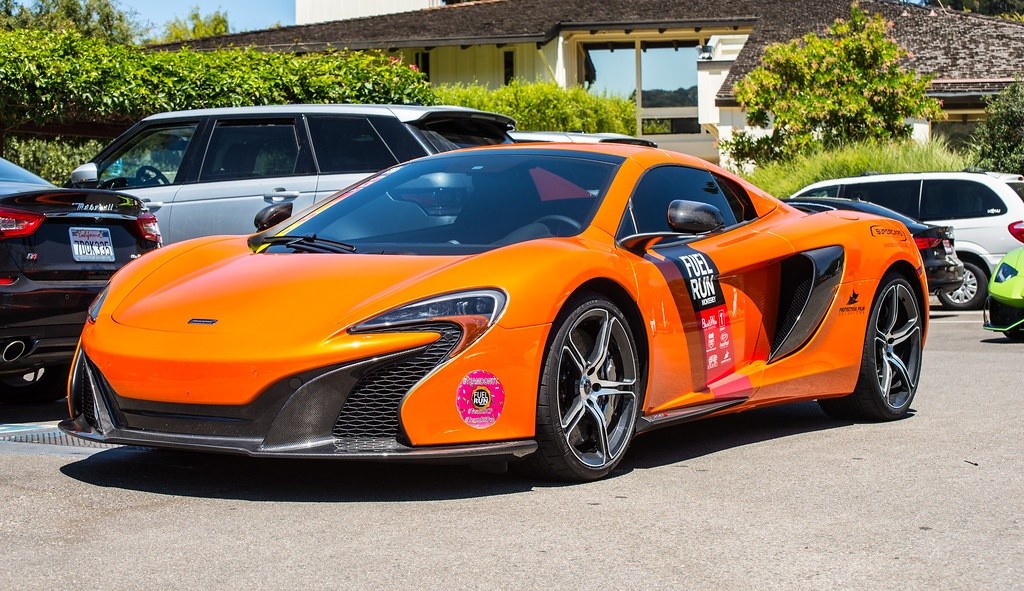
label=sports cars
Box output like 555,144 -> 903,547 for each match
60,147 -> 934,485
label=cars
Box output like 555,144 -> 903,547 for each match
508,132 -> 658,147
982,245 -> 1024,343
790,168 -> 1024,311
778,195 -> 965,297
61,103 -> 515,249
0,155 -> 164,402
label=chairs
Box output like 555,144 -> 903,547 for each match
452,196 -> 537,243
217,144 -> 257,174
